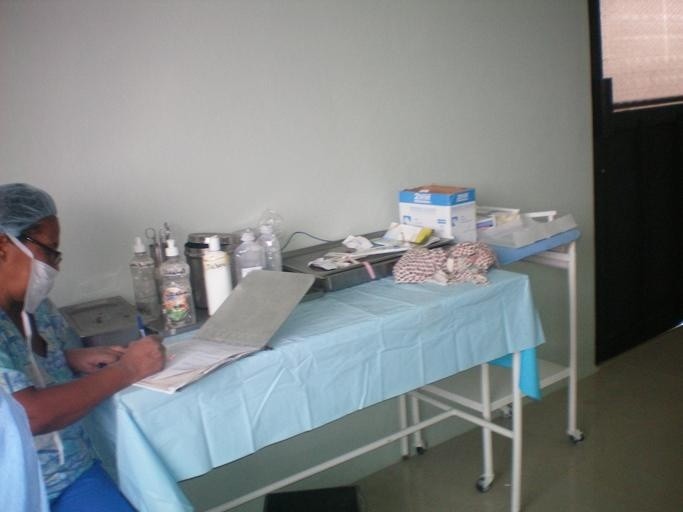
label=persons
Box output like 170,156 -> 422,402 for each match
0,181 -> 165,511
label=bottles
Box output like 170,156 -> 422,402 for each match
131,223 -> 285,331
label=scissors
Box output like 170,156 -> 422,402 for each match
145,227 -> 168,262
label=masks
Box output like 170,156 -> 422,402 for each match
4,234 -> 59,313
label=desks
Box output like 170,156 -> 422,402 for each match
72,264 -> 530,511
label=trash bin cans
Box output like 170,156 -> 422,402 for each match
263,485 -> 365,512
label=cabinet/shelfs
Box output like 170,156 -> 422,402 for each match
396,204 -> 586,491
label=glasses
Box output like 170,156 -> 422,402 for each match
24,232 -> 63,268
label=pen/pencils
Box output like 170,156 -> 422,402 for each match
136,315 -> 148,338
